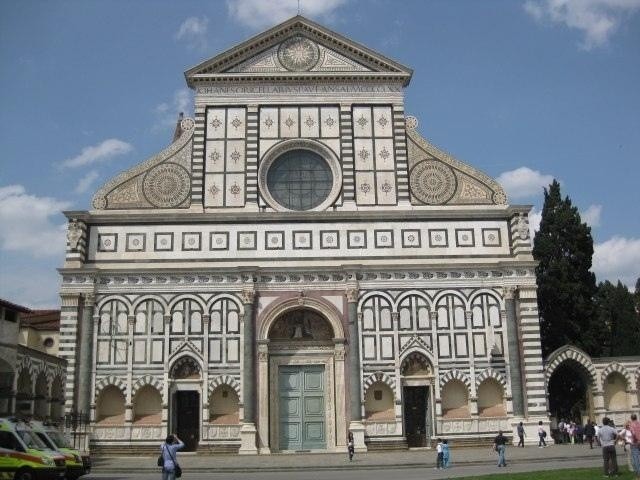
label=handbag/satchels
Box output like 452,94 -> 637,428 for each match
175,464 -> 182,478
158,456 -> 164,466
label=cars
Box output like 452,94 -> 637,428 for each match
0,415 -> 91,480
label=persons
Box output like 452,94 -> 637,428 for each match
517,422 -> 524,448
346,432 -> 354,462
441,439 -> 450,470
493,431 -> 509,467
433,438 -> 443,470
551,413 -> 640,478
161,434 -> 185,480
537,421 -> 548,448
